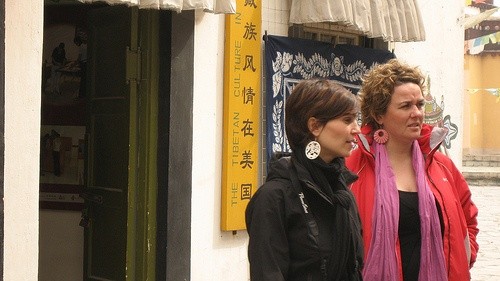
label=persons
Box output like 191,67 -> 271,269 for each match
245,79 -> 366,281
345,60 -> 479,280
